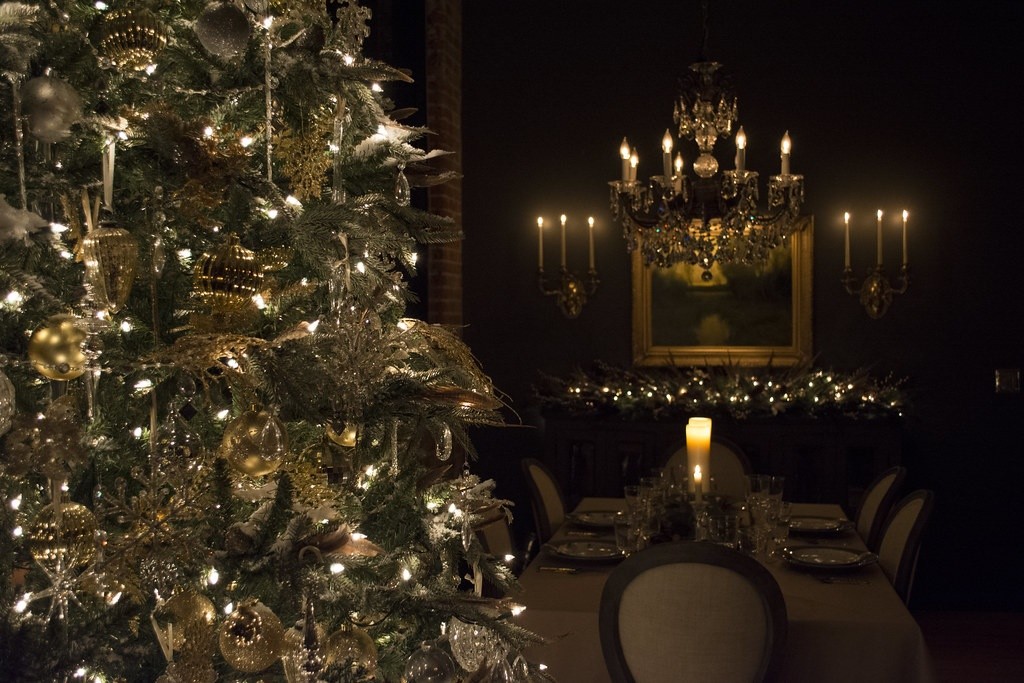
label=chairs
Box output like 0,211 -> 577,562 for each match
875,489 -> 935,608
599,540 -> 788,683
520,457 -> 566,545
461,473 -> 516,598
855,466 -> 907,547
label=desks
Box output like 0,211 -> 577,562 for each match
540,402 -> 902,505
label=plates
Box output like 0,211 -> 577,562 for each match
780,514 -> 855,534
550,538 -> 627,563
565,509 -> 626,529
775,546 -> 878,574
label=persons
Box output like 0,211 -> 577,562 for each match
674,219 -> 726,286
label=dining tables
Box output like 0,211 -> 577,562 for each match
502,497 -> 936,683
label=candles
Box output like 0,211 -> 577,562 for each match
685,416 -> 713,494
588,217 -> 595,268
902,209 -> 910,265
560,214 -> 566,266
876,208 -> 884,264
537,217 -> 544,266
844,212 -> 851,266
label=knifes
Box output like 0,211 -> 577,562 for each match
539,566 -> 606,575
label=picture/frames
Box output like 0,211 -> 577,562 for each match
631,216 -> 812,367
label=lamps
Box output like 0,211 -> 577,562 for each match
608,0 -> 805,281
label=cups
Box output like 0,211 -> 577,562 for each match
614,467 -> 792,566
